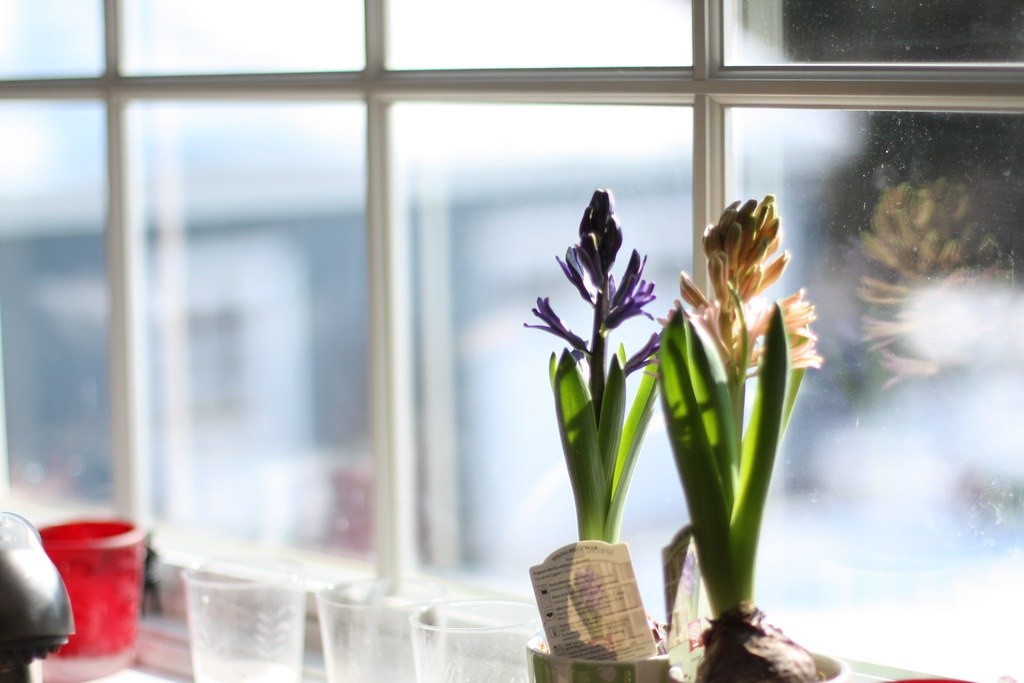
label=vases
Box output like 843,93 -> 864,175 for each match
527,645 -> 673,682
668,651 -> 849,683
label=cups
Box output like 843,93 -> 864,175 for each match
316,574 -> 447,683
37,515 -> 147,683
408,600 -> 543,683
179,557 -> 308,683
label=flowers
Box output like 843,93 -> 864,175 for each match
654,193 -> 823,683
848,172 -> 1005,389
522,187 -> 660,543
